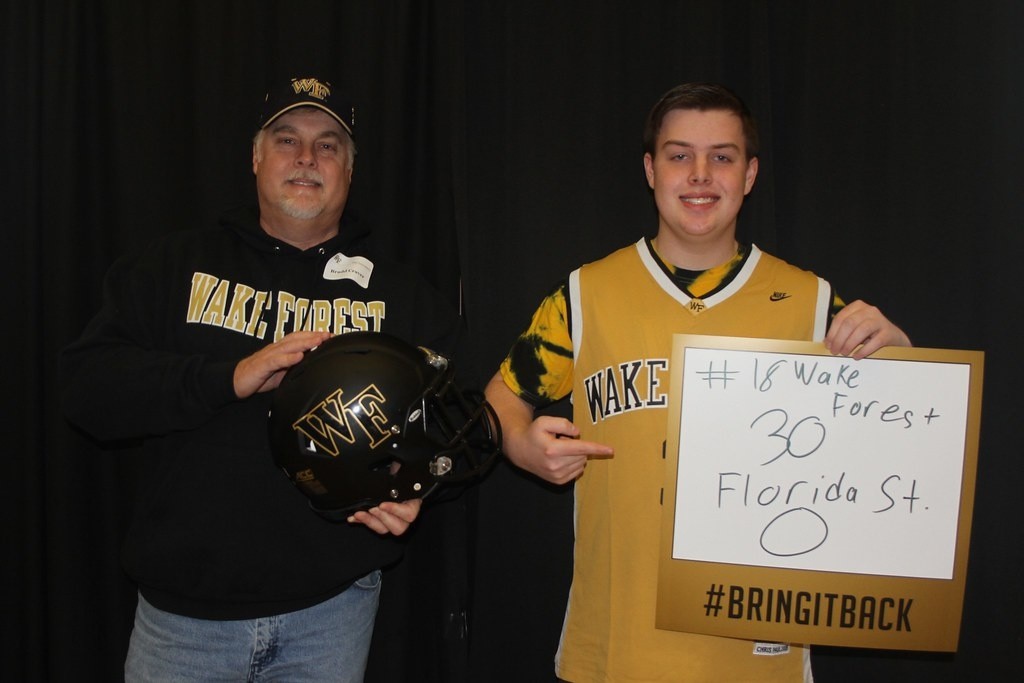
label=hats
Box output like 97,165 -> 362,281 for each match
258,73 -> 354,139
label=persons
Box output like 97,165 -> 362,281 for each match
485,87 -> 914,683
45,75 -> 465,683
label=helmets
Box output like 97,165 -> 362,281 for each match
264,332 -> 503,519
